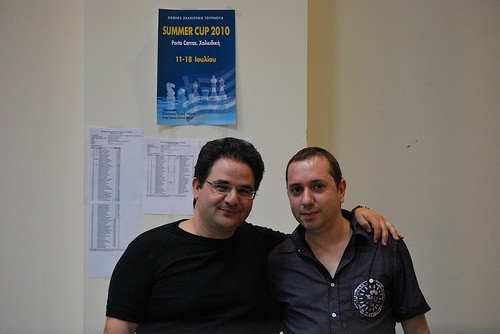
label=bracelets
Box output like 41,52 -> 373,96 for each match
350,204 -> 370,221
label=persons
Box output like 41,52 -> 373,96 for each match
104,136 -> 405,334
270,146 -> 434,334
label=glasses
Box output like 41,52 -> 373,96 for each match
201,178 -> 256,200
